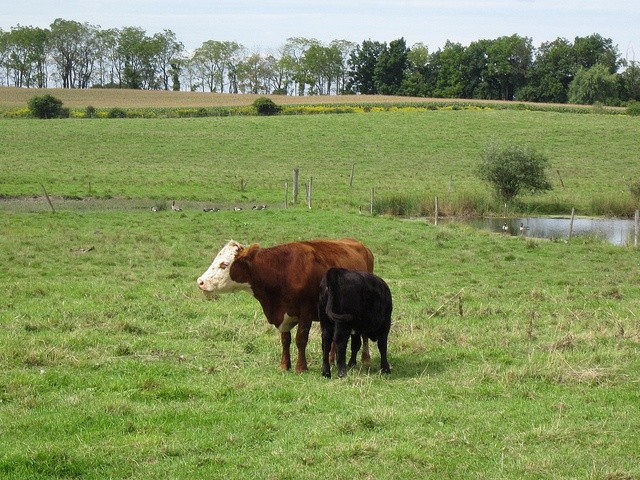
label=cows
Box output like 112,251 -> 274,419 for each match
317,267 -> 392,377
196,235 -> 374,372
